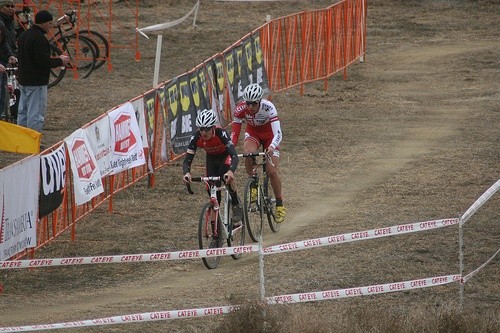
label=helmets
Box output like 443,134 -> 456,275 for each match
242,84 -> 264,101
196,109 -> 217,127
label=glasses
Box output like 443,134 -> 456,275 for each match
6,5 -> 14,8
198,127 -> 211,132
245,101 -> 257,105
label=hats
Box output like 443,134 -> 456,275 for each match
0,0 -> 13,8
34,10 -> 53,24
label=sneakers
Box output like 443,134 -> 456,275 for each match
251,188 -> 257,199
232,199 -> 242,222
275,205 -> 286,223
209,241 -> 218,248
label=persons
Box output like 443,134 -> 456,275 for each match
230,83 -> 286,223
17,9 -> 70,146
0,0 -> 21,123
183,109 -> 243,249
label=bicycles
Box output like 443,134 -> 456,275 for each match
3,4 -> 110,122
183,174 -> 247,270
234,149 -> 283,241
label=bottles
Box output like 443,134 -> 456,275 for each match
58,53 -> 68,70
